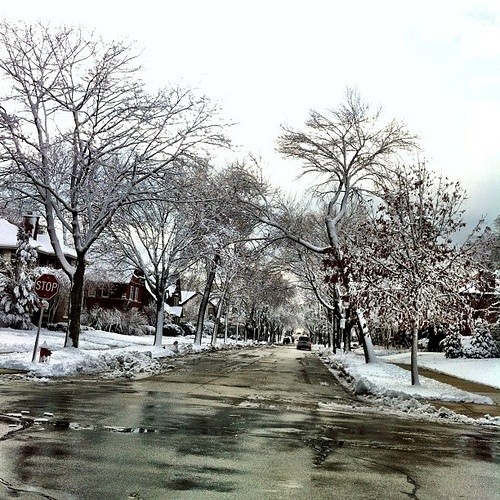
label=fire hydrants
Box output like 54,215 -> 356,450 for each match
40,340 -> 51,363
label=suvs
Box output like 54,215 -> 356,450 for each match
297,337 -> 311,351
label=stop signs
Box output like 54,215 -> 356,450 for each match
30,273 -> 60,300
